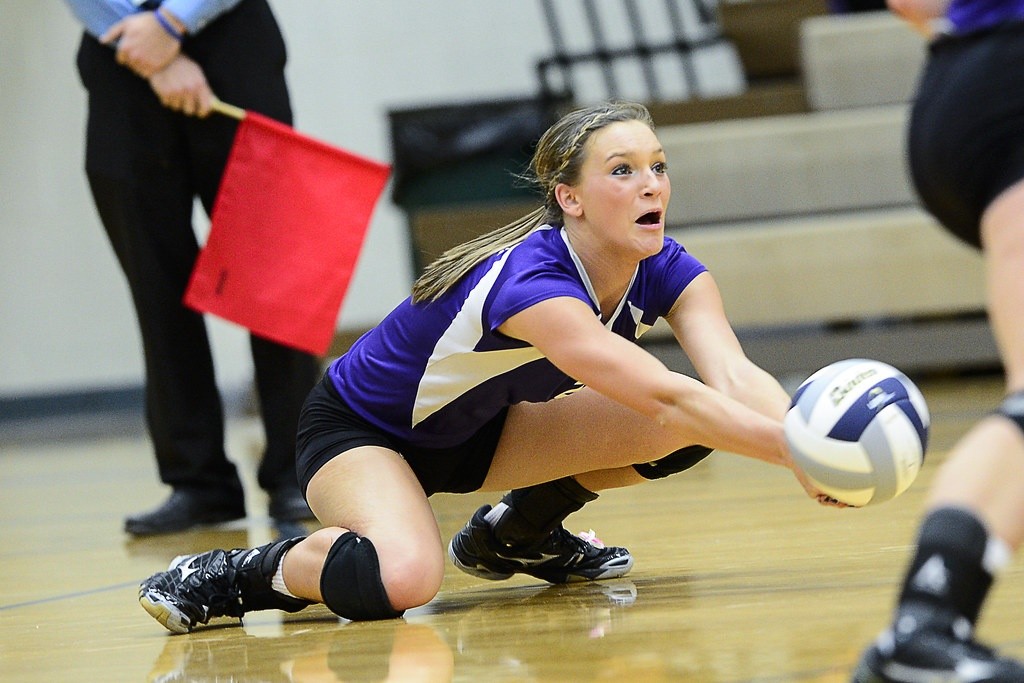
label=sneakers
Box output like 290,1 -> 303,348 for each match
138,535 -> 244,634
853,627 -> 1024,683
447,504 -> 634,585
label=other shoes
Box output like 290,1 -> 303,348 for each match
124,488 -> 247,539
266,479 -> 314,523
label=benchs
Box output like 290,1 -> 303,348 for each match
629,12 -> 987,338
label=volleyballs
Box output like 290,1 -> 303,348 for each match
785,359 -> 932,506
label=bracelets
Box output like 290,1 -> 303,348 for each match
152,11 -> 187,43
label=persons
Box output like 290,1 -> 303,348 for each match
850,1 -> 1024,683
138,102 -> 864,633
60,0 -> 326,537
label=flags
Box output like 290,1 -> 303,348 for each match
184,112 -> 390,358
